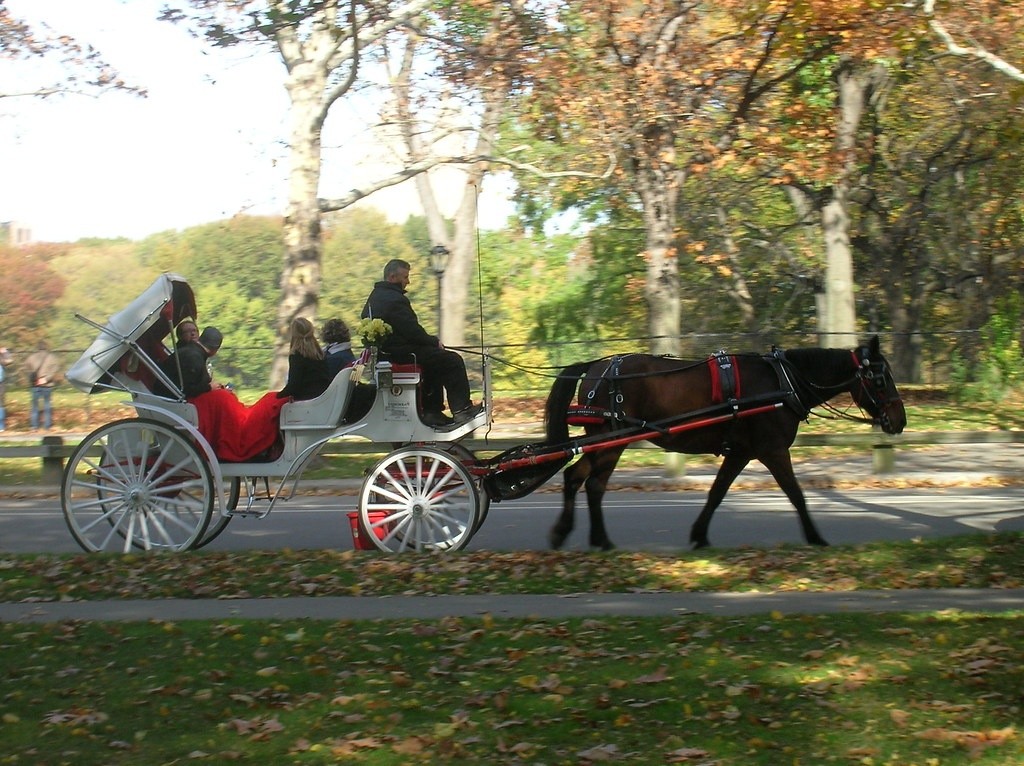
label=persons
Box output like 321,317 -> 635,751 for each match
0,347 -> 14,430
319,318 -> 358,377
22,340 -> 60,430
361,259 -> 483,426
264,316 -> 329,461
152,320 -> 248,460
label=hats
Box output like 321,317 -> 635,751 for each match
199,326 -> 222,351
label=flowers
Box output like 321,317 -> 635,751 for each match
354,317 -> 393,348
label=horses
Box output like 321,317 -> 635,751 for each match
542,335 -> 907,554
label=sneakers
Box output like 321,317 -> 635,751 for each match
453,403 -> 482,423
422,412 -> 453,425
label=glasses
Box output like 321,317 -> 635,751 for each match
180,328 -> 199,335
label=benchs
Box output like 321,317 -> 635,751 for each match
119,342 -> 199,443
378,352 -> 424,417
279,349 -> 369,427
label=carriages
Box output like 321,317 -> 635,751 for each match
59,275 -> 909,561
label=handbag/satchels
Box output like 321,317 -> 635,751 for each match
31,372 -> 37,381
